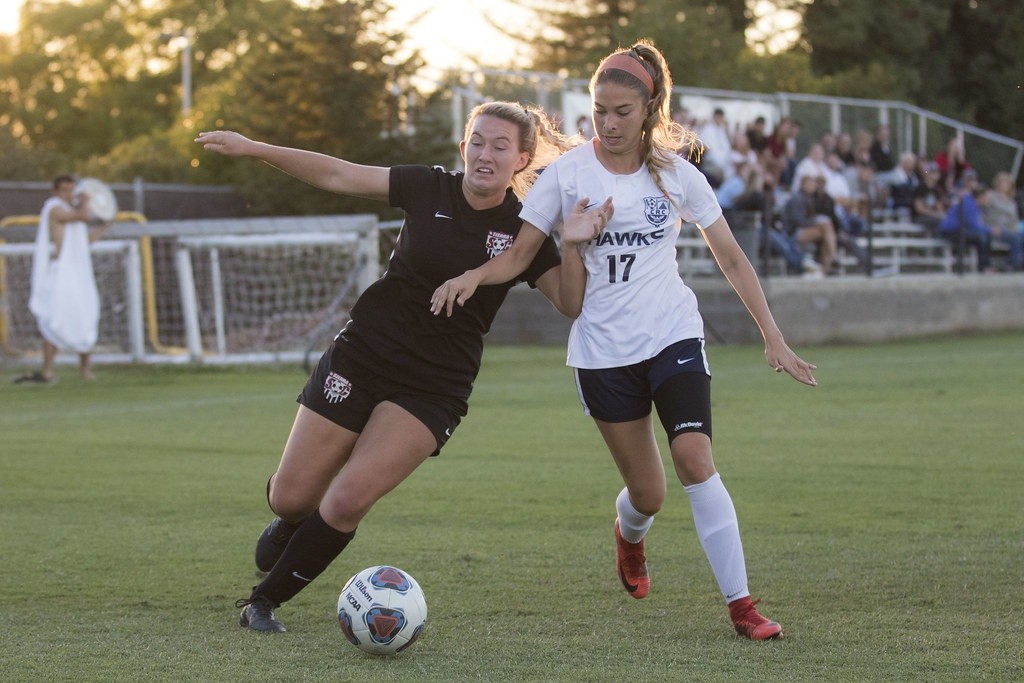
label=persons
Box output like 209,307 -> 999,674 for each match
28,176 -> 115,382
195,103 -> 614,635
575,115 -> 588,143
430,46 -> 818,639
675,108 -> 1024,278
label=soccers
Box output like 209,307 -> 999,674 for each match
336,564 -> 430,657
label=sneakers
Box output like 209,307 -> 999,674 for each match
254,518 -> 300,572
615,517 -> 649,598
235,586 -> 286,633
728,595 -> 782,641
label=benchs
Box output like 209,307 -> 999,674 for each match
676,201 -> 1011,277
